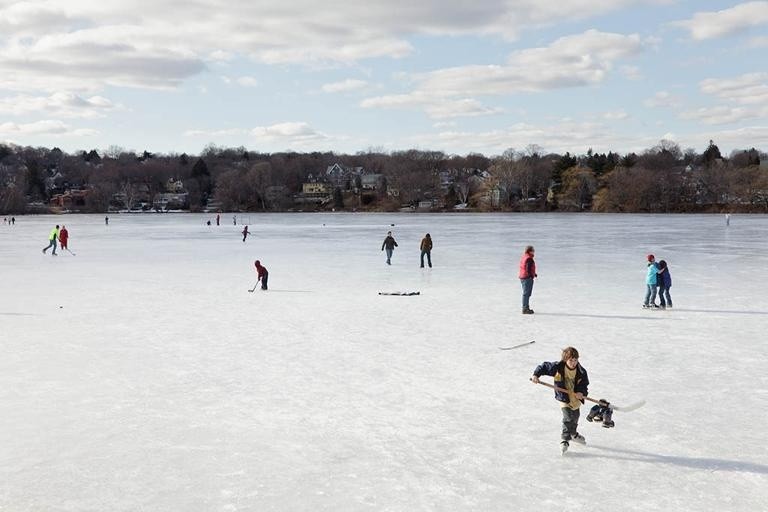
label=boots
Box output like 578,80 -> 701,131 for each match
585,398 -> 615,428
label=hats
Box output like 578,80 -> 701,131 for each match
647,254 -> 654,261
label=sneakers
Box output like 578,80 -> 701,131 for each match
560,438 -> 570,456
570,432 -> 587,446
42,249 -> 46,254
522,308 -> 534,315
642,304 -> 673,308
52,253 -> 57,256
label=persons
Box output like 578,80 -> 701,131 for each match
641,253 -> 666,309
254,259 -> 269,291
381,230 -> 399,265
241,226 -> 251,243
59,226 -> 69,249
233,215 -> 237,226
725,211 -> 731,227
41,224 -> 62,257
658,259 -> 674,308
531,345 -> 589,454
419,232 -> 434,269
216,213 -> 220,225
518,245 -> 537,315
